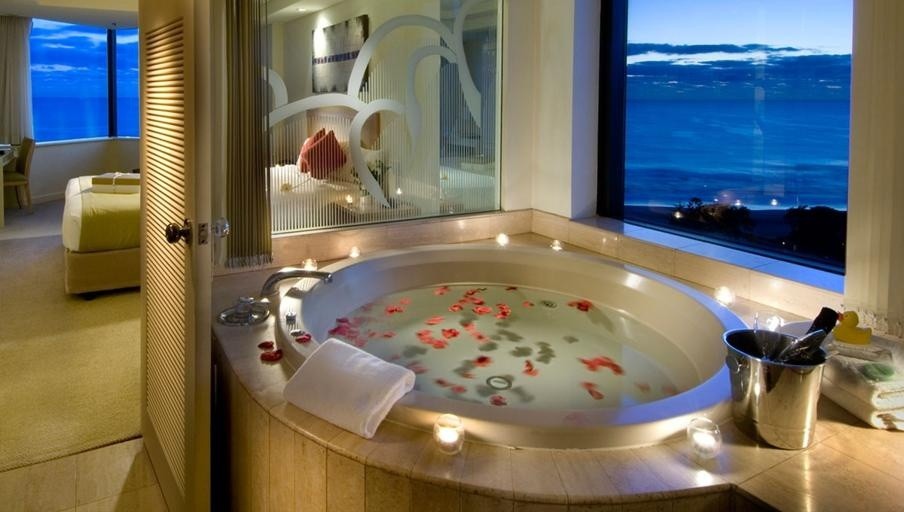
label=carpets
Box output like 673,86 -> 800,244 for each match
0,291 -> 143,471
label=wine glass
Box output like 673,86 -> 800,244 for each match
753,312 -> 782,359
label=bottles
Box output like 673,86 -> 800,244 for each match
806,306 -> 838,346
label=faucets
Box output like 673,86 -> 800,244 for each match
261,266 -> 332,298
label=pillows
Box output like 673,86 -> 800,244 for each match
296,127 -> 384,190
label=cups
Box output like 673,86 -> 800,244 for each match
433,413 -> 465,455
713,286 -> 734,310
774,329 -> 840,364
685,416 -> 722,463
301,258 -> 317,272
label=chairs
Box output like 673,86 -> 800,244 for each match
4,137 -> 35,214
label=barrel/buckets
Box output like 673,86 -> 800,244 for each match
723,328 -> 830,451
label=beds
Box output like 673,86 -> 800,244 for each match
62,106 -> 379,301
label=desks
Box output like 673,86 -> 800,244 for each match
0,144 -> 24,229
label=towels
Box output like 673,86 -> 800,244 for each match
282,338 -> 416,440
778,319 -> 904,431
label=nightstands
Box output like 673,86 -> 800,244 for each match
329,197 -> 421,224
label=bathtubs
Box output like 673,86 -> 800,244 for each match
275,244 -> 755,451
